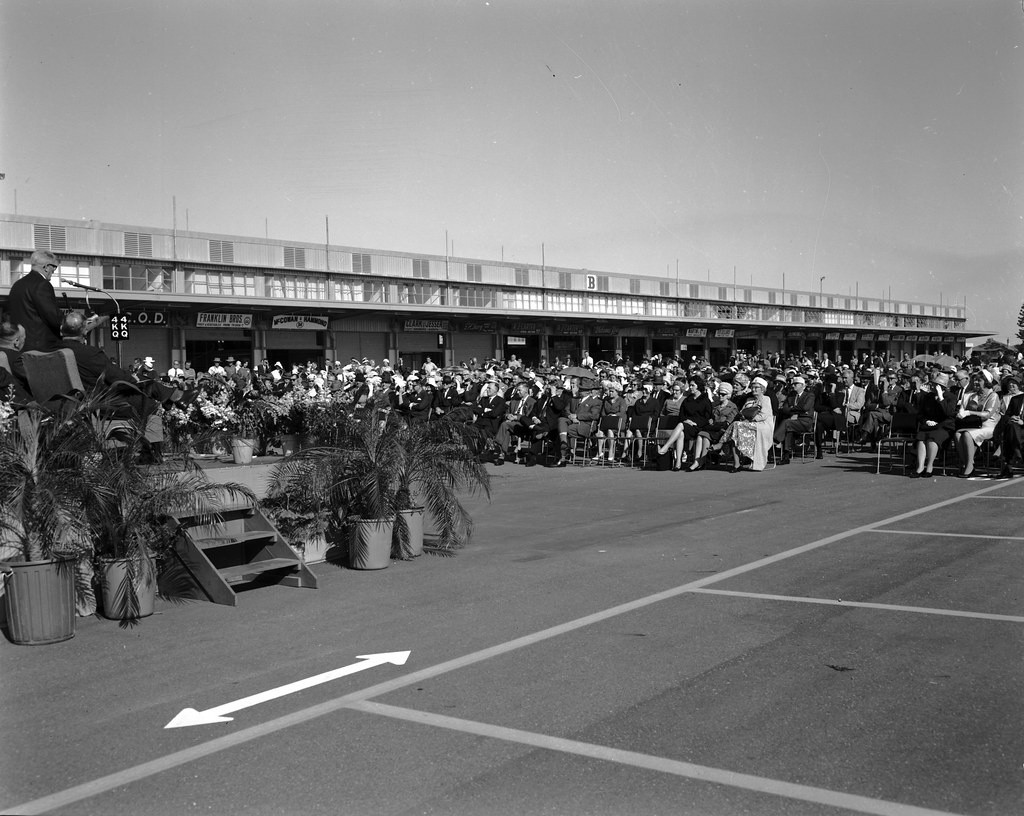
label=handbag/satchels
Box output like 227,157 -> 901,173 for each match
919,424 -> 938,431
741,402 -> 761,419
629,414 -> 652,433
963,415 -> 982,428
599,415 -> 620,431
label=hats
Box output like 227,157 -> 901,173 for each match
440,376 -> 454,383
141,356 -> 294,380
353,373 -> 366,382
733,365 -> 913,396
931,363 -> 1024,390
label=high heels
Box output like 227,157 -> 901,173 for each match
910,468 -> 935,478
957,462 -> 977,478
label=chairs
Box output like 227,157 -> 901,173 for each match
507,402 -> 1023,478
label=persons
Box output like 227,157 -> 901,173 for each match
107,356 -> 1024,480
9,246 -> 66,353
0,321 -> 34,398
63,310 -> 205,467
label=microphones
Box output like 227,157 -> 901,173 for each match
60,278 -> 100,292
61,292 -> 74,313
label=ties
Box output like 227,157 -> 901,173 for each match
790,395 -> 800,421
516,399 -> 582,415
1020,407 -> 1024,420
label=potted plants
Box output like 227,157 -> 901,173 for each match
1,363 -> 148,646
219,394 -> 492,571
48,385 -> 263,627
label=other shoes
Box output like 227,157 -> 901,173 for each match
816,437 -> 880,459
995,472 -> 1014,479
771,443 -> 777,448
495,447 -> 741,473
150,452 -> 163,465
776,458 -> 790,465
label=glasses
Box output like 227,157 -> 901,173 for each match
47,264 -> 57,270
718,393 -> 727,396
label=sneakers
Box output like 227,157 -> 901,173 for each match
162,384 -> 202,411
